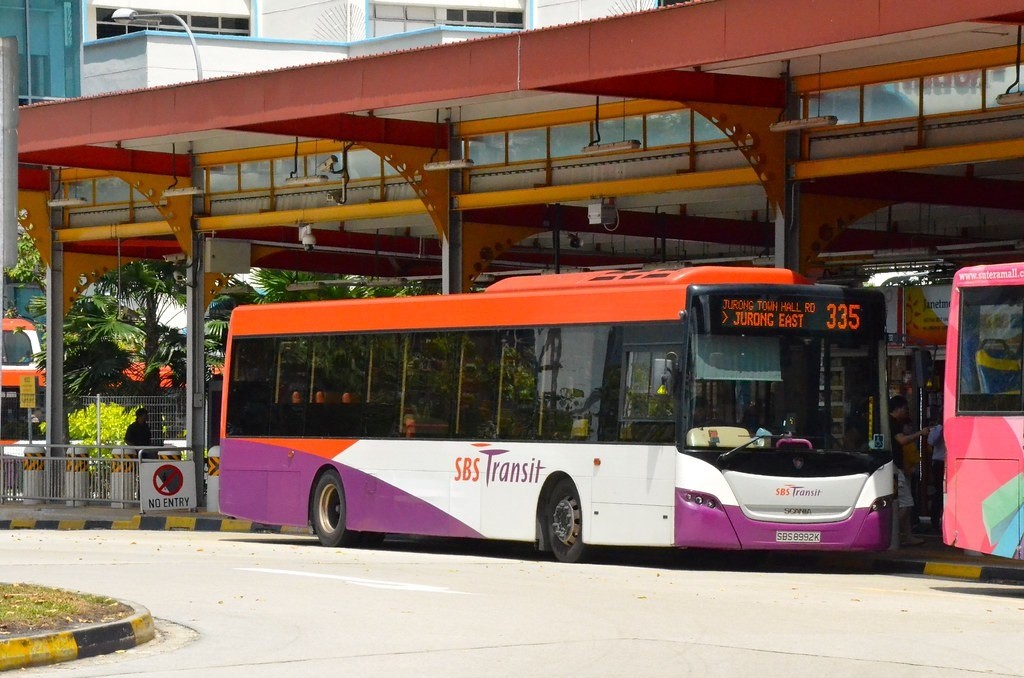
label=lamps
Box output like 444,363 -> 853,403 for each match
162,143 -> 203,197
580,95 -> 641,155
47,167 -> 87,207
996,25 -> 1024,105
285,136 -> 329,185
769,55 -> 838,133
424,107 -> 474,171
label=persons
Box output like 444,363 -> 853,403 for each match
125,408 -> 152,446
890,396 -> 930,546
927,422 -> 945,496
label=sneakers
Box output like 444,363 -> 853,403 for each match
899,533 -> 925,546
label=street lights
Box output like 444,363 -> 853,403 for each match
111,7 -> 204,81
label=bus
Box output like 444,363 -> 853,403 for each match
941,261 -> 1024,559
218,266 -> 893,563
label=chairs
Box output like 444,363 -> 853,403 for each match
291,391 -> 362,403
973,337 -> 1023,395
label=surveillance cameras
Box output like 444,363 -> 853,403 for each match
302,235 -> 316,251
162,251 -> 185,262
317,154 -> 338,173
173,270 -> 184,282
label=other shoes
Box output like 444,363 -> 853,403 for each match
135,408 -> 150,415
